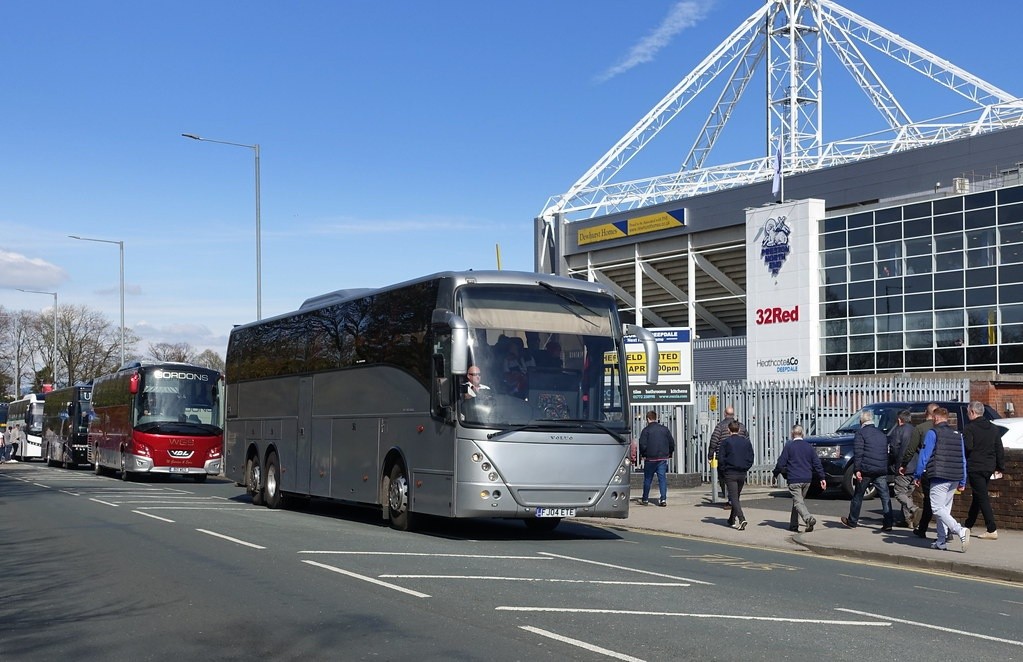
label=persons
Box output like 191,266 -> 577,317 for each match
899,404 -> 953,543
963,401 -> 1005,540
913,408 -> 971,553
612,414 -> 636,465
707,407 -> 749,510
637,411 -> 675,507
0,424 -> 21,464
460,366 -> 491,401
718,420 -> 754,531
888,410 -> 923,529
487,333 -> 563,402
772,424 -> 826,532
841,411 -> 893,534
144,398 -> 165,416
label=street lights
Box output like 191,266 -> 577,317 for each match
16,288 -> 58,390
182,134 -> 262,321
68,235 -> 125,367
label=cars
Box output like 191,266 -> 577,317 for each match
801,401 -> 1002,500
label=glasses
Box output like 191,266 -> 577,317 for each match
469,372 -> 482,376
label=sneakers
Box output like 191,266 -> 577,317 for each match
930,541 -> 947,550
958,527 -> 970,552
977,530 -> 997,539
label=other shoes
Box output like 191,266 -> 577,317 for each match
913,529 -> 926,537
881,527 -> 892,531
841,517 -> 854,529
937,533 -> 953,541
805,517 -> 816,532
738,521 -> 747,530
789,526 -> 798,531
913,509 -> 923,526
637,498 -> 648,506
659,502 -> 666,506
727,519 -> 736,528
895,521 -> 913,528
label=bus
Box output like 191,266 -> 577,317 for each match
223,270 -> 659,535
40,384 -> 92,468
5,393 -> 48,462
86,361 -> 226,481
0,403 -> 9,434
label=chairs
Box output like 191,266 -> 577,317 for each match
189,415 -> 201,424
